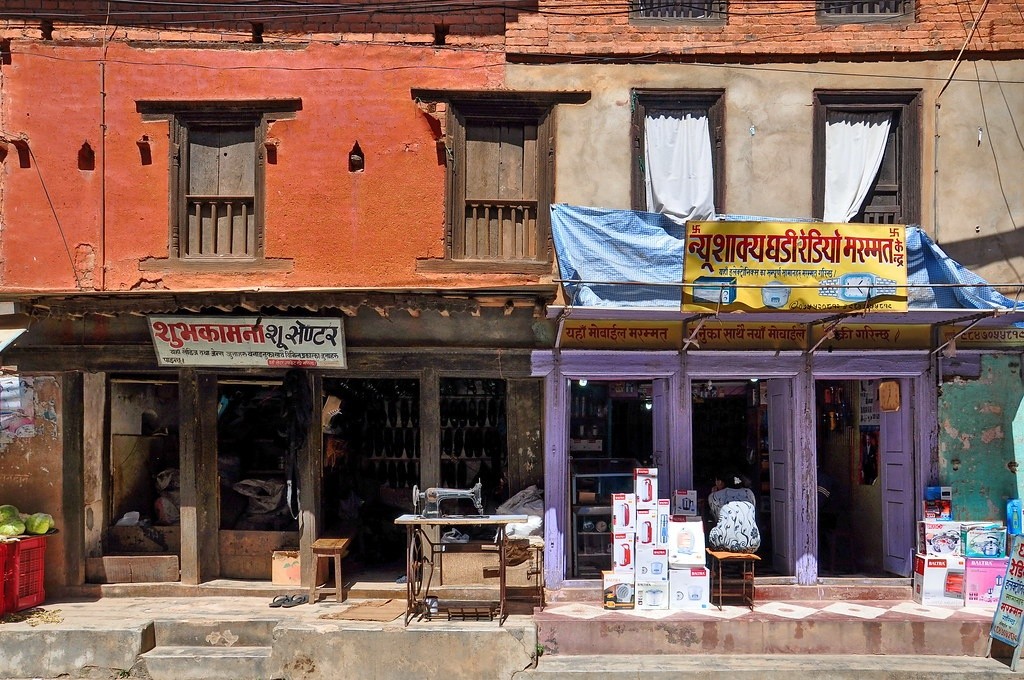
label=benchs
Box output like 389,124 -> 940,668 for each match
309,539 -> 351,603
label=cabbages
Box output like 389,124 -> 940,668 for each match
0,504 -> 55,536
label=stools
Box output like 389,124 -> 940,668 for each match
706,549 -> 763,611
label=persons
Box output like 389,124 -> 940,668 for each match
707,468 -> 761,586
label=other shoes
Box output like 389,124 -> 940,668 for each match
367,389 -> 502,495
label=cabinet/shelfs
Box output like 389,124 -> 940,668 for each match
572,457 -> 642,578
570,415 -> 608,438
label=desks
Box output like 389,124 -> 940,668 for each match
393,514 -> 529,626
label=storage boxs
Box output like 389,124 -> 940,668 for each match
913,488 -> 1024,607
578,492 -> 596,504
602,467 -> 710,611
0,535 -> 46,619
271,547 -> 330,588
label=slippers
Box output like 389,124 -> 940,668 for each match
269,594 -> 292,607
282,594 -> 309,607
